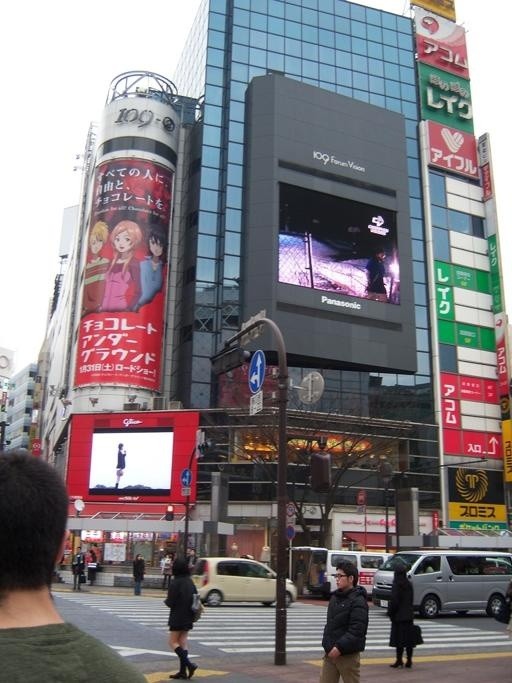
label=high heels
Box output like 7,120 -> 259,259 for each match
391,660 -> 403,668
406,659 -> 412,667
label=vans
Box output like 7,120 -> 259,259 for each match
373,547 -> 512,617
286,545 -> 407,600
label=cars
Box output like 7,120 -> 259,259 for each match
192,556 -> 298,611
486,558 -> 512,572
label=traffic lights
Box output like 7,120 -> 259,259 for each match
198,438 -> 216,454
166,506 -> 174,520
210,345 -> 251,372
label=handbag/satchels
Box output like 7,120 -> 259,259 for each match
415,624 -> 423,645
190,594 -> 202,622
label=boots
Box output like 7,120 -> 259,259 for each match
169,649 -> 188,679
175,646 -> 198,678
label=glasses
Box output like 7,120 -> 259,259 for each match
335,573 -> 349,577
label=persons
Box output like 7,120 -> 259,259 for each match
160,551 -> 174,589
163,557 -> 202,679
387,563 -> 415,667
187,547 -> 199,570
366,252 -> 392,300
131,554 -> 146,597
71,543 -> 103,589
116,443 -> 127,489
318,559 -> 368,682
83,220 -> 166,316
293,554 -> 309,595
0,451 -> 148,683
